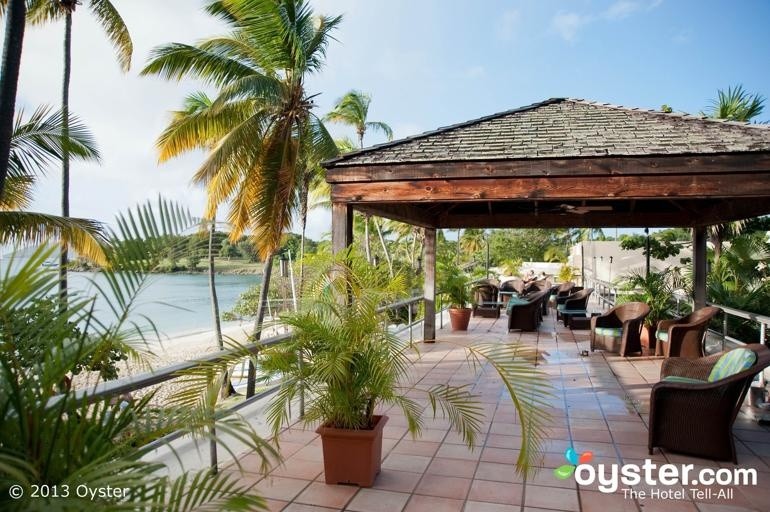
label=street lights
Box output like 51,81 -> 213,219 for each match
278,254 -> 288,333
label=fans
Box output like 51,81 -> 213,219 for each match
545,202 -> 612,215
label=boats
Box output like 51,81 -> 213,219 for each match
43,261 -> 59,266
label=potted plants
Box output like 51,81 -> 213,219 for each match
162,240 -> 483,492
441,263 -> 473,330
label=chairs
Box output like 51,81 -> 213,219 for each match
471,277 -> 594,332
589,301 -> 770,466
